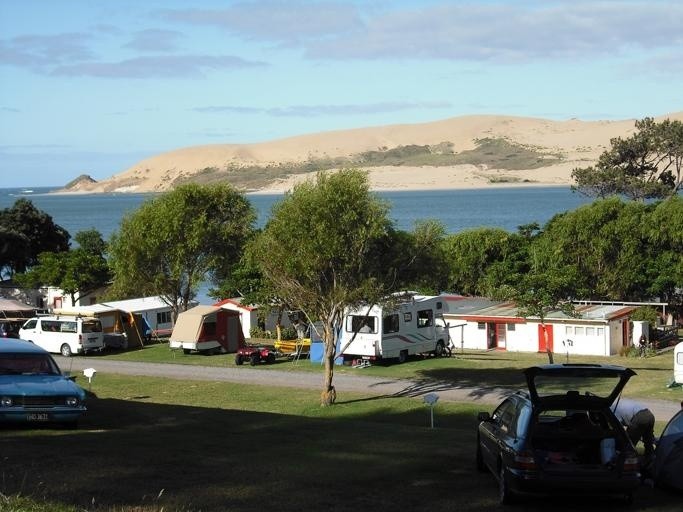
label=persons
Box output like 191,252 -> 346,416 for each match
637,333 -> 647,359
610,398 -> 657,460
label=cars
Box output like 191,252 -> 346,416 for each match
476,362 -> 643,506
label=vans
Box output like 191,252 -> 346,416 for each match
16,314 -> 103,357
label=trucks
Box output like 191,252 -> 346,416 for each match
337,290 -> 451,365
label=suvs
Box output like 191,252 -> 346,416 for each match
234,343 -> 276,366
0,334 -> 88,426
